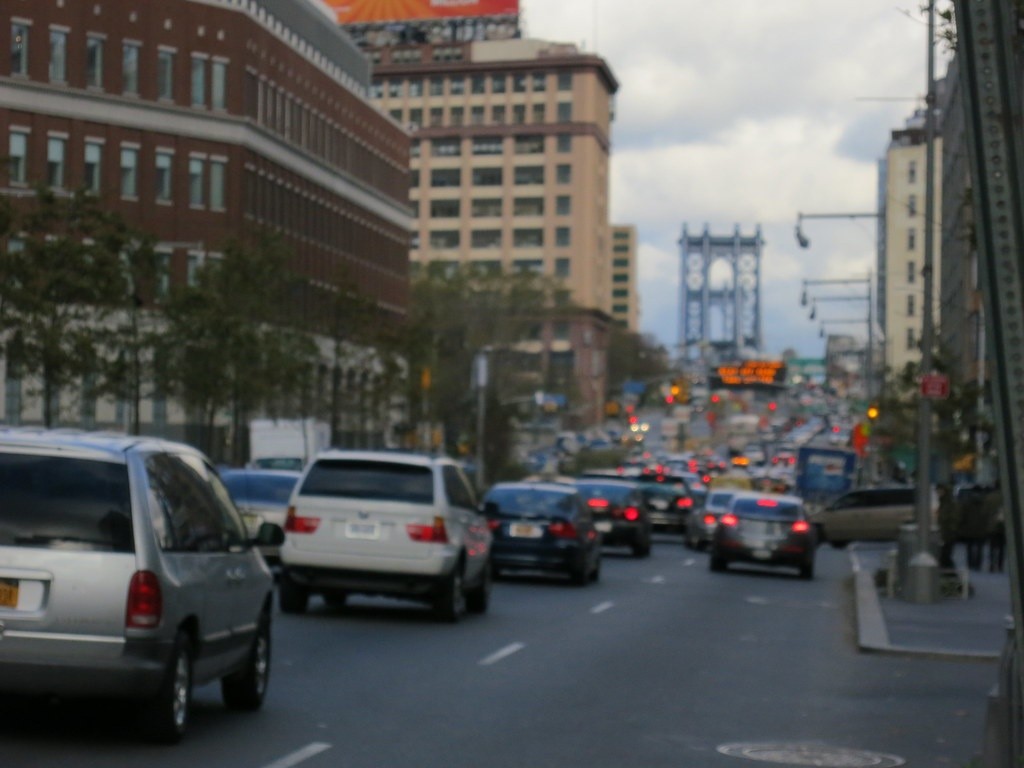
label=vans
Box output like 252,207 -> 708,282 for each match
805,489 -> 931,549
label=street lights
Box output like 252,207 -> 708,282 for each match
905,0 -> 942,612
791,207 -> 887,490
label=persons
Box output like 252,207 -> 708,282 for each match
934,483 -> 956,569
967,492 -> 1006,576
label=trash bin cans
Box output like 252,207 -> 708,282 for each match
896,524 -> 940,582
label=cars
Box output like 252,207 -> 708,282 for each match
488,482 -> 603,585
593,415 -> 868,552
217,465 -> 309,570
565,481 -> 651,558
709,493 -> 821,581
282,451 -> 496,624
0,425 -> 285,747
